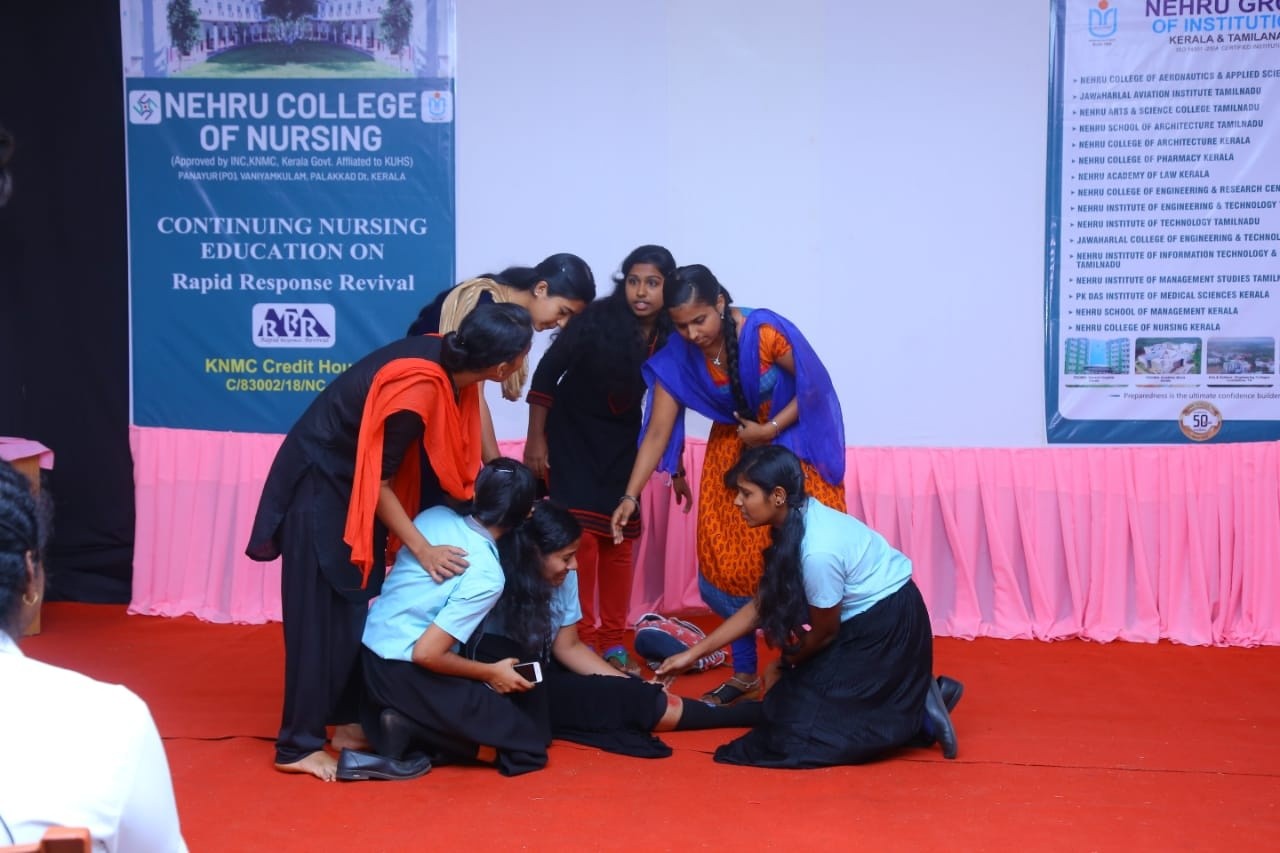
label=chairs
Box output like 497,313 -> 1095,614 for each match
0,826 -> 93,853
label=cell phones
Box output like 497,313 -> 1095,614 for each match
514,661 -> 543,684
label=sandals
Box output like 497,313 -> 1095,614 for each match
698,676 -> 760,705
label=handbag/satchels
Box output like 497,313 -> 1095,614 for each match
634,611 -> 727,672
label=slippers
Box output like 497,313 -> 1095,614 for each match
603,647 -> 642,677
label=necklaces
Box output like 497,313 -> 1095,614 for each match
706,334 -> 726,365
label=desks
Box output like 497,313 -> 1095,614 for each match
5,454 -> 43,638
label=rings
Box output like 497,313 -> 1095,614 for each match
736,428 -> 743,433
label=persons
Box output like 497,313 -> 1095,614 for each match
0,456 -> 188,852
245,245 -> 962,783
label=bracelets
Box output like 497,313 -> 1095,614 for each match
770,420 -> 780,438
670,470 -> 686,479
618,495 -> 641,517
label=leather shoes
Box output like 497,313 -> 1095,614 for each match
337,745 -> 434,780
925,674 -> 963,758
